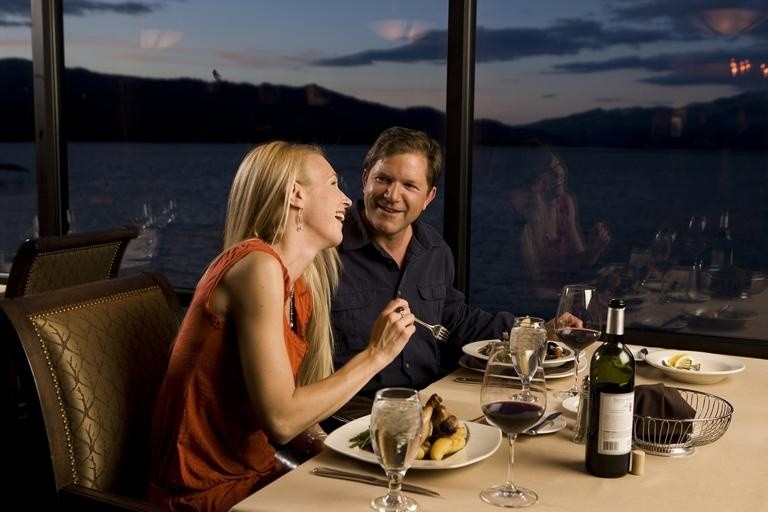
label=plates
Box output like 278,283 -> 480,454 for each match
644,349 -> 747,386
620,346 -> 664,364
457,333 -> 588,381
484,405 -> 566,436
596,207 -> 767,331
325,410 -> 503,473
562,394 -> 583,413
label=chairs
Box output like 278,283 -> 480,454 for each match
4,225 -> 139,298
0,269 -> 299,512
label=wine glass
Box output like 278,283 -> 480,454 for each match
478,283 -> 602,507
370,385 -> 423,512
131,196 -> 180,256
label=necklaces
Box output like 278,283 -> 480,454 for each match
286,286 -> 302,330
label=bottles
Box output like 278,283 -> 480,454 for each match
579,298 -> 636,479
570,373 -> 590,444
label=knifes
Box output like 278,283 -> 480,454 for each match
528,410 -> 564,435
314,465 -> 442,498
452,375 -> 544,394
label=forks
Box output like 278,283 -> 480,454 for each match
411,317 -> 452,343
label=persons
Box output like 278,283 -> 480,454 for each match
141,140 -> 420,512
461,141 -> 613,336
521,153 -> 614,304
293,127 -> 587,434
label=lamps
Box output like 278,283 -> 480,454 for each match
688,6 -> 766,42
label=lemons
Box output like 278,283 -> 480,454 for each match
670,354 -> 692,368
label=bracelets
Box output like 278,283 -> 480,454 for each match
303,431 -> 327,460
557,290 -> 563,299
